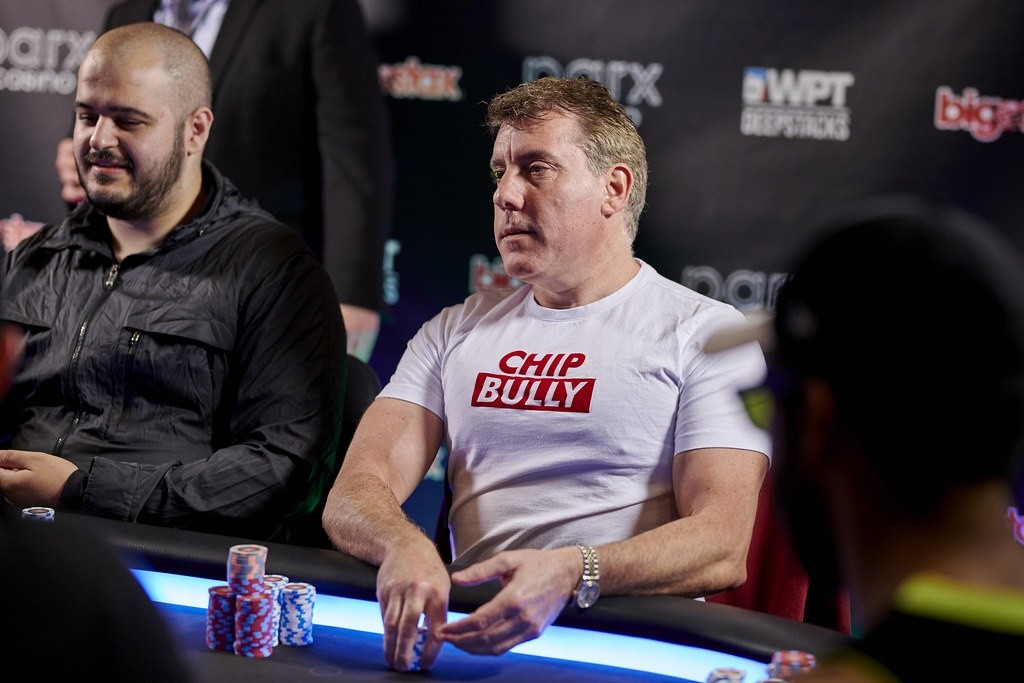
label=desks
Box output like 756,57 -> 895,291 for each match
36,509 -> 844,683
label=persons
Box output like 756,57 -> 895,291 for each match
56,0 -> 395,309
705,207 -> 1023,683
0,515 -> 183,683
320,77 -> 774,672
0,22 -> 348,530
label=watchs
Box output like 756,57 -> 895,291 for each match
569,544 -> 601,609
